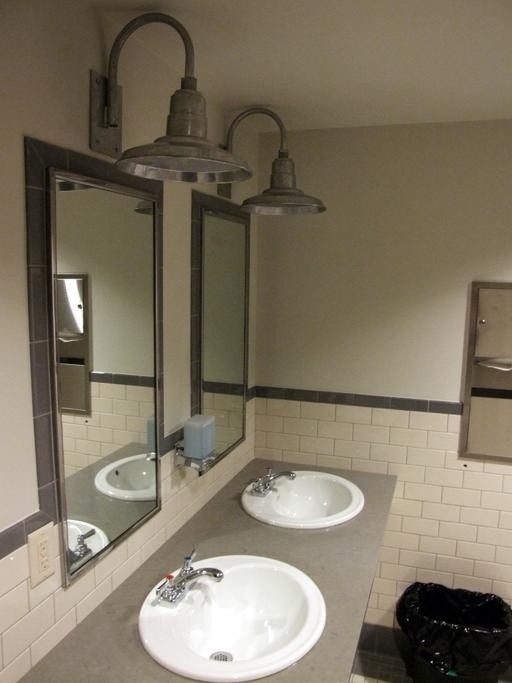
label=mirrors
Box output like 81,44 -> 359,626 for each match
189,187 -> 257,474
19,135 -> 166,589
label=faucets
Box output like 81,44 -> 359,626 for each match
146,454 -> 156,462
155,548 -> 223,610
68,529 -> 96,565
249,468 -> 296,498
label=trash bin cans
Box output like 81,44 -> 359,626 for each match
396,582 -> 512,683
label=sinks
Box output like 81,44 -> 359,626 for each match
67,519 -> 110,567
240,470 -> 365,529
137,554 -> 327,683
94,452 -> 157,502
